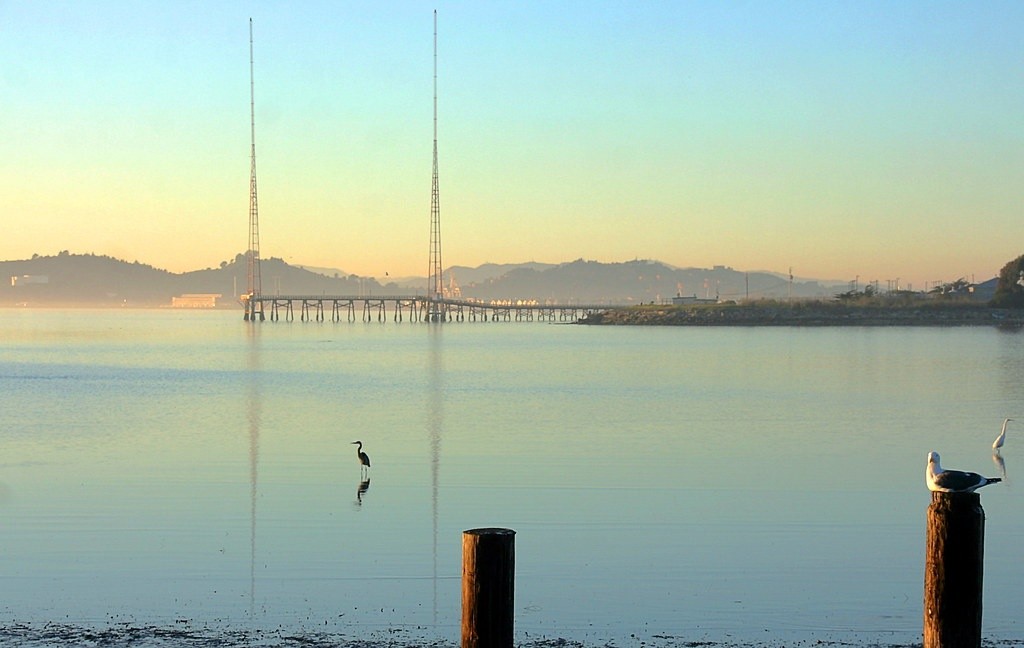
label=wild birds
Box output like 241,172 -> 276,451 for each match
350,441 -> 372,481
924,451 -> 1002,492
991,418 -> 1015,450
